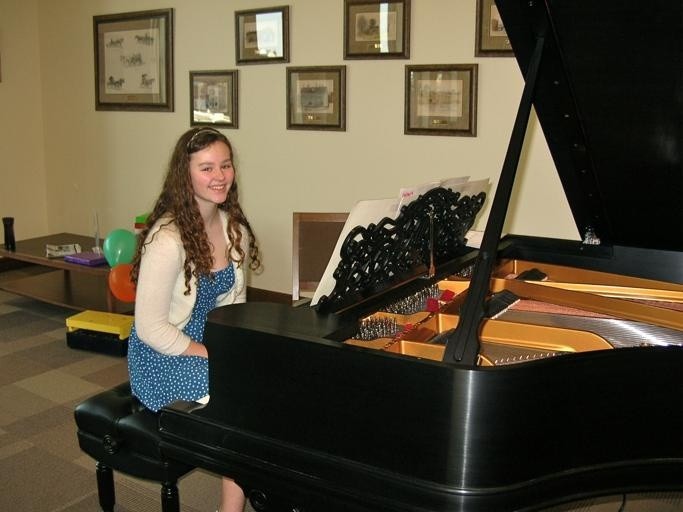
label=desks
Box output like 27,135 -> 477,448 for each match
0,233 -> 135,316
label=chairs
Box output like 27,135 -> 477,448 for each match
292,213 -> 349,301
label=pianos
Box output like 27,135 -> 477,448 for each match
158,1 -> 682,511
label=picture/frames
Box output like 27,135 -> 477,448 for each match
234,5 -> 289,65
93,8 -> 176,112
189,70 -> 237,129
404,63 -> 478,137
343,1 -> 411,60
475,1 -> 516,57
286,65 -> 345,131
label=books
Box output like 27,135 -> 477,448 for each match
65,252 -> 105,266
310,176 -> 490,304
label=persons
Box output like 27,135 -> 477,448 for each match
127,127 -> 260,512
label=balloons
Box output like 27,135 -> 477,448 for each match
108,264 -> 135,303
101,229 -> 137,267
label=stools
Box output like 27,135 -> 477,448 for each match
74,381 -> 194,512
